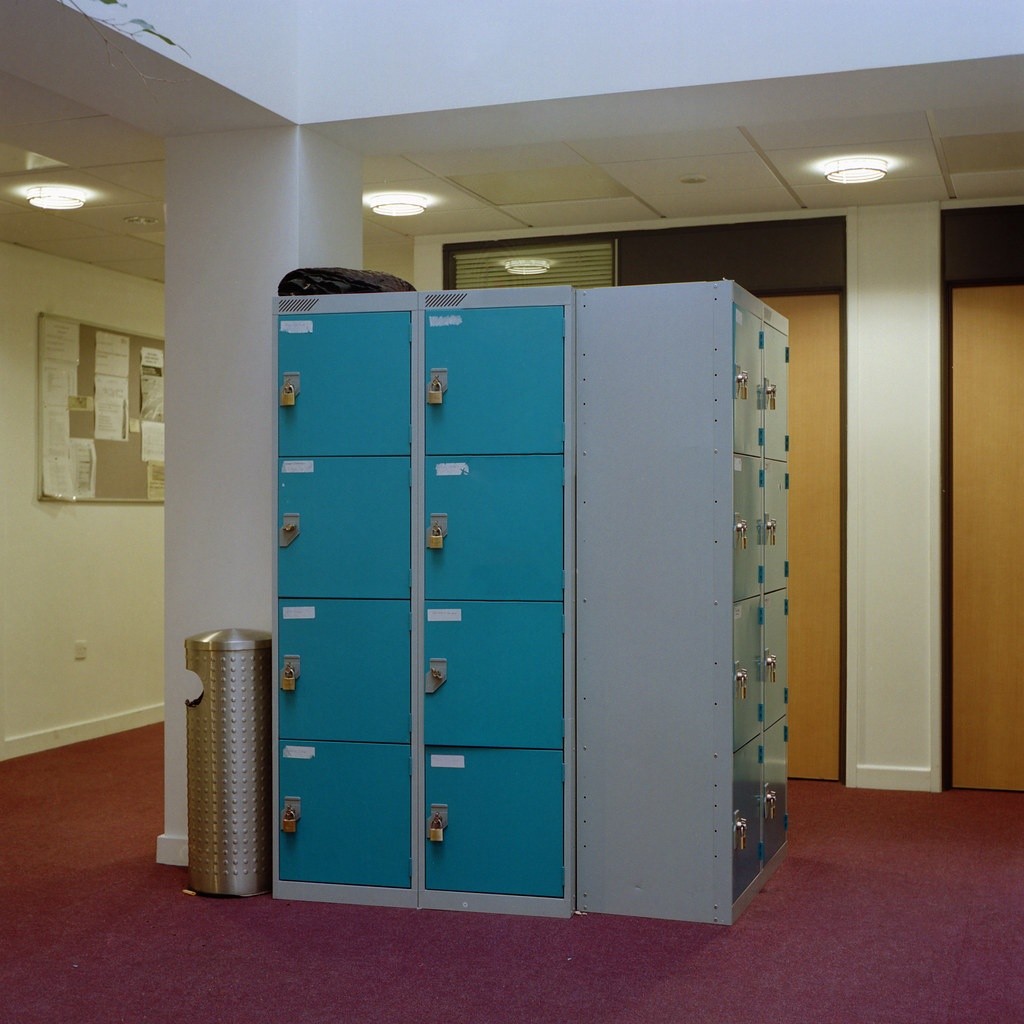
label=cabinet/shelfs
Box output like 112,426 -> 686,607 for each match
572,279 -> 762,927
415,285 -> 576,918
761,303 -> 790,885
272,291 -> 416,908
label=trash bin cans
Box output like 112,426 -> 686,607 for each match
183,628 -> 273,899
